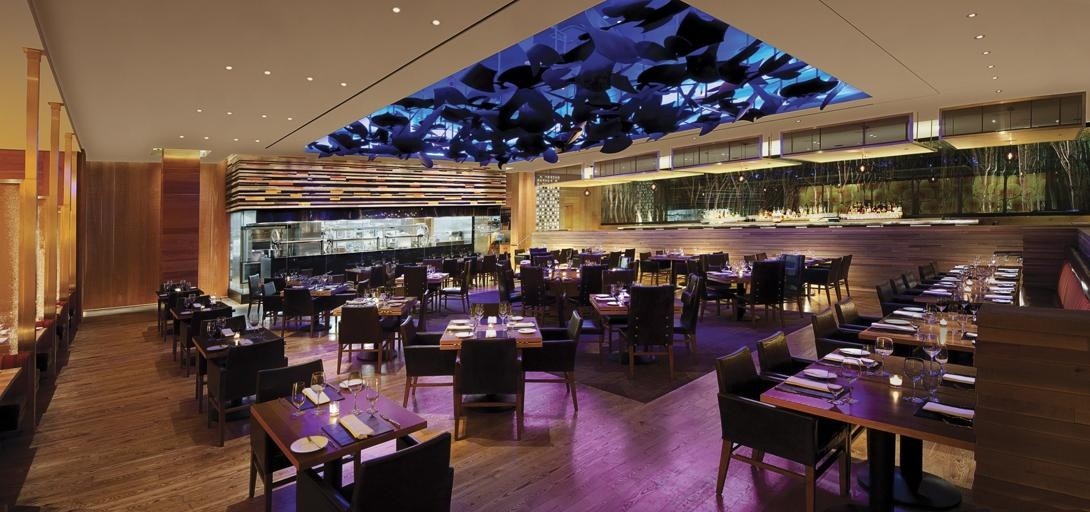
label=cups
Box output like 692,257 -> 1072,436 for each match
328,400 -> 340,416
361,364 -> 376,387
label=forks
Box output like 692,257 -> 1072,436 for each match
943,418 -> 973,429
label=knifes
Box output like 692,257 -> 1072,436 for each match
306,434 -> 322,450
380,412 -> 400,426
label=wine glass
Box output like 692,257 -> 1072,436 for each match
720,259 -> 754,274
310,371 -> 326,415
292,381 -> 306,417
827,253 -> 997,403
363,287 -> 387,306
163,279 -> 259,338
348,370 -> 362,415
363,373 -> 380,414
471,301 -> 511,330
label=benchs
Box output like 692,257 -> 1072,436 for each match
1023,243 -> 1090,313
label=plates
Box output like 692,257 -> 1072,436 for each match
340,379 -> 363,387
289,435 -> 328,452
355,296 -> 404,306
206,344 -> 230,350
994,291 -> 1011,294
993,299 -> 1010,303
452,315 -> 537,337
1002,275 -> 1016,277
803,369 -> 837,379
597,293 -> 619,306
